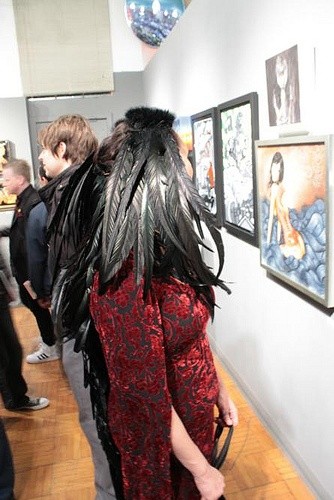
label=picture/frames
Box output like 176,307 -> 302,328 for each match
217,92 -> 259,241
254,135 -> 334,308
191,107 -> 222,229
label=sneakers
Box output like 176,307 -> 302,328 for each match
7,397 -> 49,410
26,341 -> 62,364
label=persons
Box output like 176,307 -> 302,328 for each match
26,166 -> 46,309
47,108 -> 238,500
0,160 -> 62,364
273,54 -> 297,126
38,115 -> 116,500
0,280 -> 49,412
